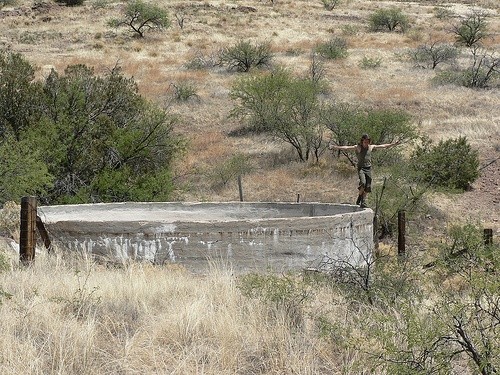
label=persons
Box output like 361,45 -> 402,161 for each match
329,134 -> 401,208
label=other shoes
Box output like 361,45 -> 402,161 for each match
356,202 -> 360,205
361,202 -> 369,208
